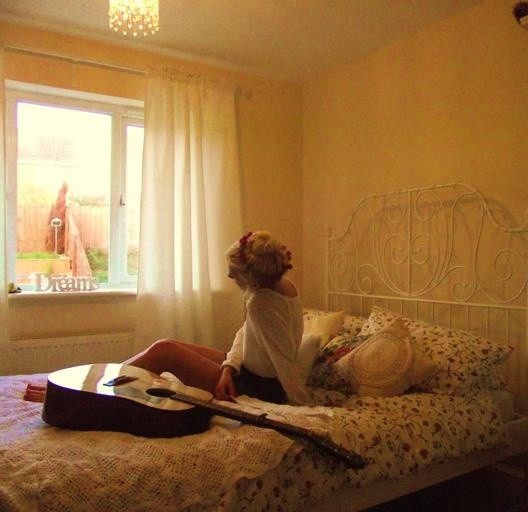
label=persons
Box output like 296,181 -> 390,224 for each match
21,229 -> 317,409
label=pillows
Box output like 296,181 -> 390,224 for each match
294,305 -> 515,407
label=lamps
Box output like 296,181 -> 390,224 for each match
108,0 -> 160,38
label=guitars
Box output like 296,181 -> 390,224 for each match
41,364 -> 367,470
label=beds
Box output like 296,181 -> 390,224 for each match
1,183 -> 528,512
